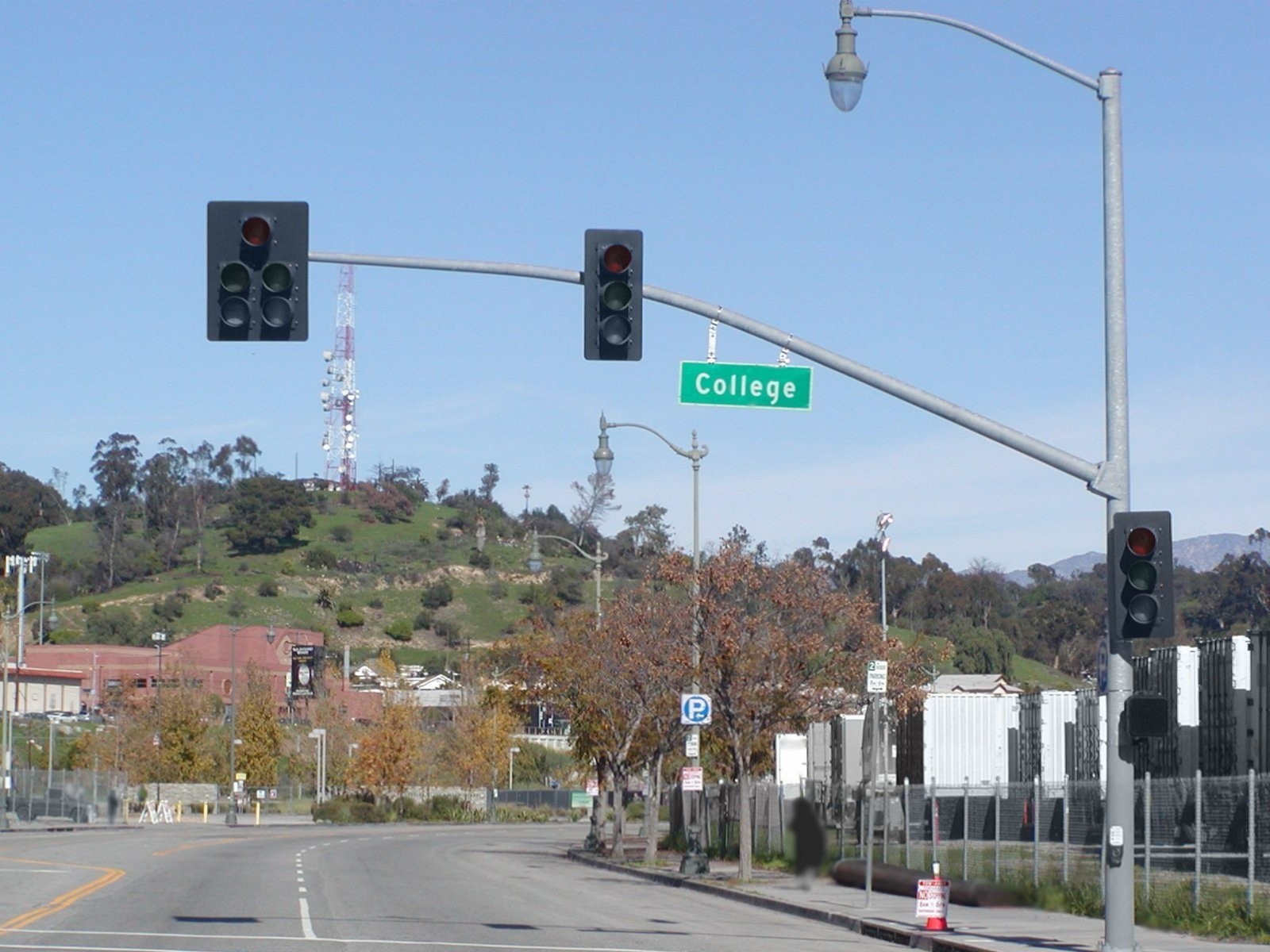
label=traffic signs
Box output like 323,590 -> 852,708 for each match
680,693 -> 712,725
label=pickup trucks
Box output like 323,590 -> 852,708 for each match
6,786 -> 102,825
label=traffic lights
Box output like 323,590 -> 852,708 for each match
1113,512 -> 1175,641
206,201 -> 309,342
1125,695 -> 1170,738
585,228 -> 643,360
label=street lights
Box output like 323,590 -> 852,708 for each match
3,550 -> 51,668
309,728 -> 328,806
592,412 -> 710,879
918,859 -> 956,933
151,631 -> 168,814
225,618 -> 275,827
0,594 -> 58,828
348,742 -> 359,760
823,0 -> 1135,952
509,747 -> 521,790
